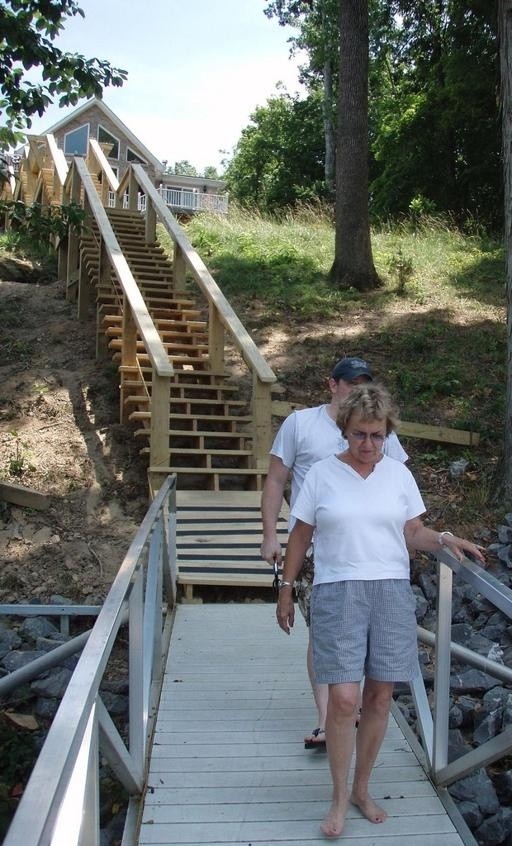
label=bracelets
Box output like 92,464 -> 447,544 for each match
278,580 -> 292,590
439,531 -> 454,548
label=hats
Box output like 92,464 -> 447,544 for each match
331,357 -> 374,383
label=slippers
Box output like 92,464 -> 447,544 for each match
305,727 -> 326,750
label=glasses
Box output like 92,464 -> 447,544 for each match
346,425 -> 390,443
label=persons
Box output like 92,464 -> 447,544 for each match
259,358 -> 413,752
271,382 -> 486,838
